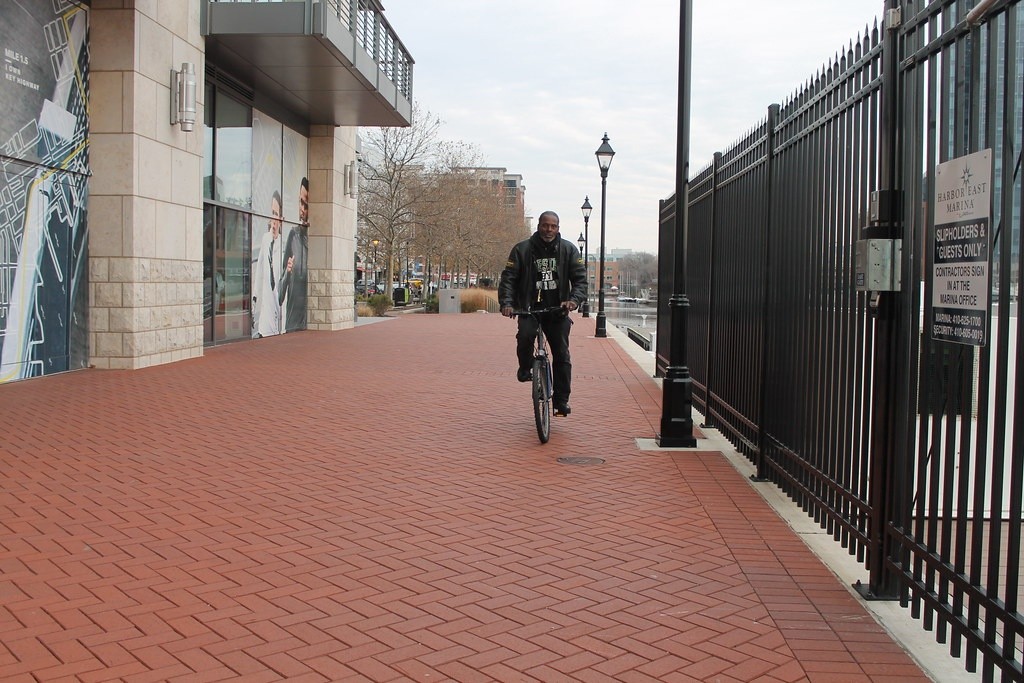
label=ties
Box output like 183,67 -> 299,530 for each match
268,238 -> 275,290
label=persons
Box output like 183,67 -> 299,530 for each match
498,211 -> 588,414
252,190 -> 288,339
278,177 -> 309,333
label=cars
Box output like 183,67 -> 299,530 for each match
355,280 -> 374,293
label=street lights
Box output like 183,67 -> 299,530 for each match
371,239 -> 381,294
577,232 -> 585,313
594,132 -> 616,338
580,196 -> 593,319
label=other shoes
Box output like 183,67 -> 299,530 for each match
553,400 -> 571,414
517,356 -> 536,382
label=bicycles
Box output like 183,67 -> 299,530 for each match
510,305 -> 574,444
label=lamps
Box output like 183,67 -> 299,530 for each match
344,160 -> 359,199
171,62 -> 197,132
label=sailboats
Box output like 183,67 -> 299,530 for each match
616,268 -> 650,304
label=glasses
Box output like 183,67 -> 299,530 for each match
300,198 -> 307,210
